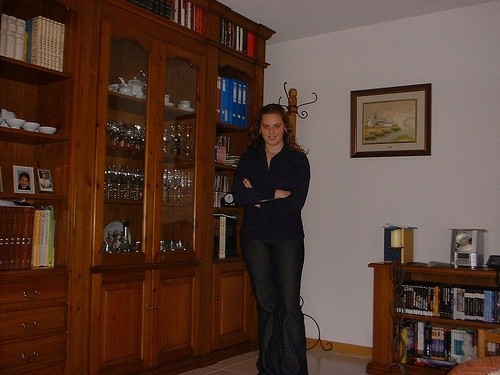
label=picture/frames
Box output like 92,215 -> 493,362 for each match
350,83 -> 431,158
38,169 -> 53,192
13,165 -> 36,194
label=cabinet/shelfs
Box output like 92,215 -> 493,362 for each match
0,0 -> 278,375
366,261 -> 500,375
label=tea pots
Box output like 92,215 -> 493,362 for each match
118,77 -> 147,100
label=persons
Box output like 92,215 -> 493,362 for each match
40,172 -> 51,188
18,172 -> 31,190
231,102 -> 310,375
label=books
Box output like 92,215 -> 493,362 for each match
395,280 -> 500,325
216,135 -> 240,167
213,214 -> 238,260
219,18 -> 257,58
128,0 -> 203,33
213,175 -> 234,208
393,318 -> 500,367
0,13 -> 65,72
0,200 -> 55,271
217,75 -> 248,129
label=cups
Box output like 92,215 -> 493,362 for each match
180,101 -> 191,108
110,84 -> 118,92
119,84 -> 131,93
165,95 -> 170,106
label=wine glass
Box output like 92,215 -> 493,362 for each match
162,124 -> 194,157
104,162 -> 143,200
162,168 -> 193,203
106,119 -> 145,150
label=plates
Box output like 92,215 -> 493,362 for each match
178,107 -> 195,111
103,221 -> 131,248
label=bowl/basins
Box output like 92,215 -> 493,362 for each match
22,123 -> 40,131
37,126 -> 57,134
4,118 -> 26,129
